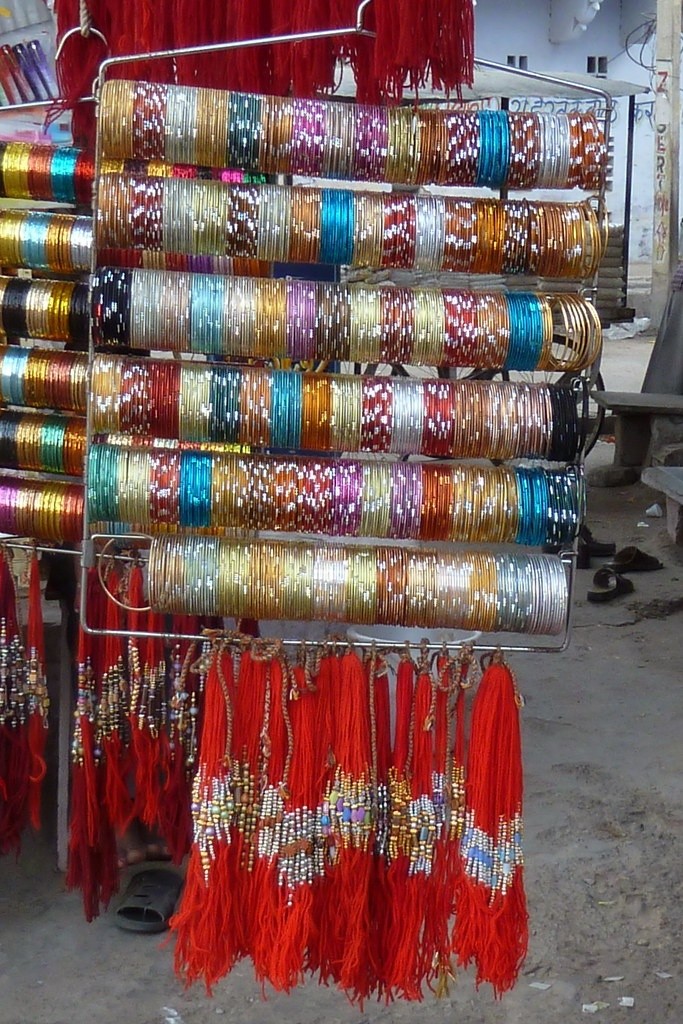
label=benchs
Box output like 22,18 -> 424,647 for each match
588,391 -> 683,487
642,467 -> 683,542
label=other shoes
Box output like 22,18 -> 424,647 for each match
543,523 -> 616,570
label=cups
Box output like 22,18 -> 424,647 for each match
646,503 -> 662,517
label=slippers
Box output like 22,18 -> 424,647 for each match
602,546 -> 663,573
587,565 -> 634,602
115,870 -> 186,930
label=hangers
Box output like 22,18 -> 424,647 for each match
0,0 -> 610,652
